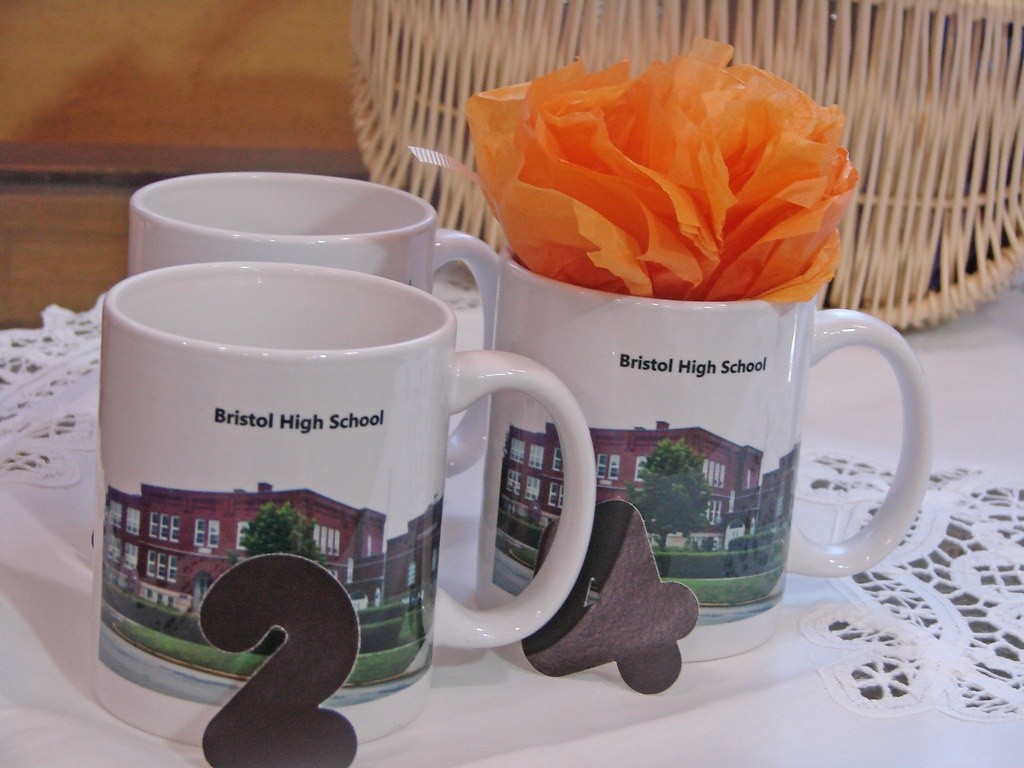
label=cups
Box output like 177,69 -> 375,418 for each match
99,262 -> 597,750
476,236 -> 932,664
126,171 -> 499,476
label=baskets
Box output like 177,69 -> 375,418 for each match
348,0 -> 1024,332
